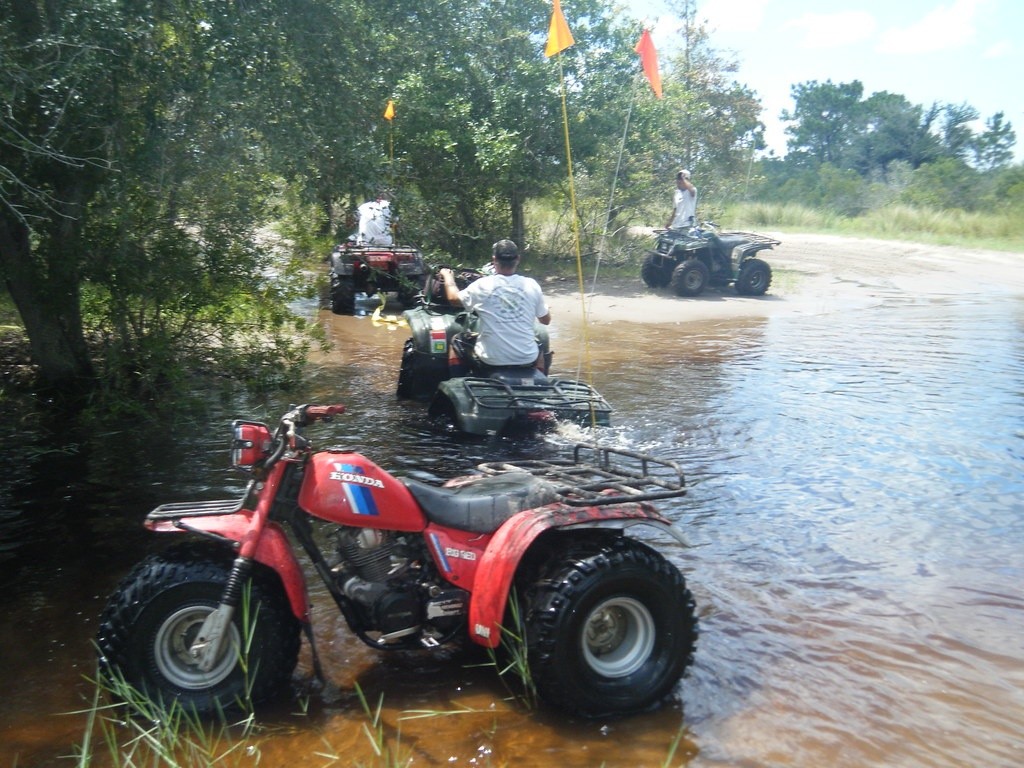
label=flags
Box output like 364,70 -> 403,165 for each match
544,0 -> 575,58
635,28 -> 664,101
384,100 -> 395,121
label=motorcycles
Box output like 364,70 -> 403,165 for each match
397,264 -> 613,436
95,401 -> 702,725
641,215 -> 783,296
329,227 -> 427,317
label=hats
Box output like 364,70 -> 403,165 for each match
677,169 -> 691,179
493,237 -> 519,261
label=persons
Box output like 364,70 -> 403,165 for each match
439,240 -> 551,380
356,195 -> 392,246
664,170 -> 698,235
